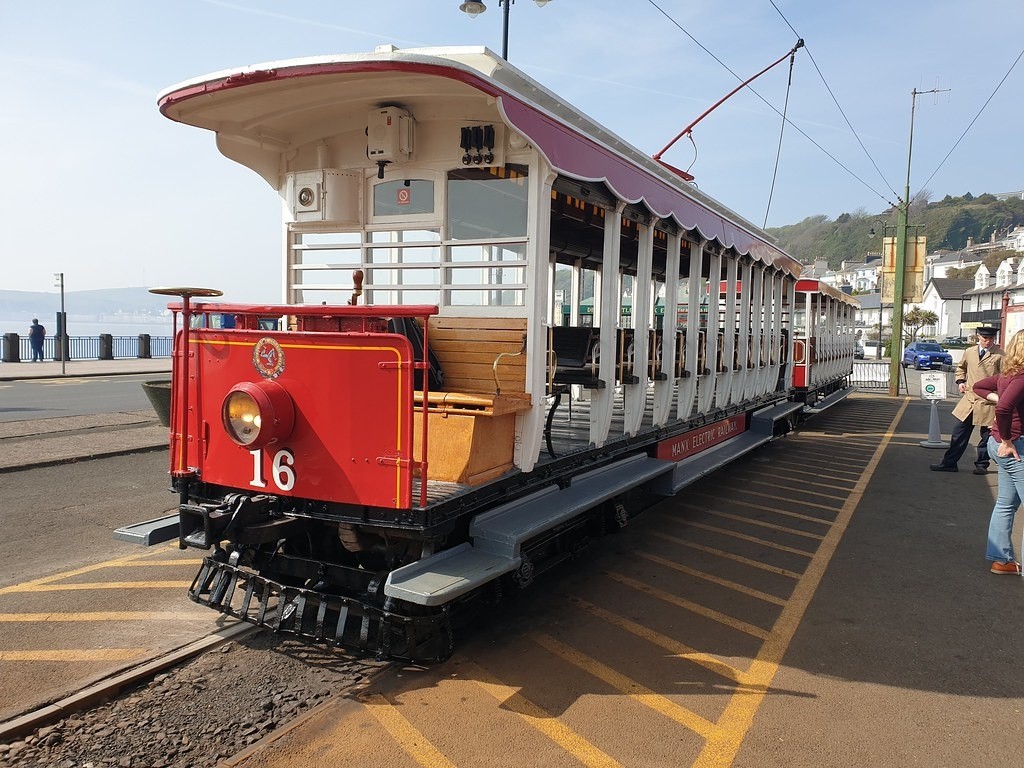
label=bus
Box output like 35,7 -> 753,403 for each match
108,41 -> 864,665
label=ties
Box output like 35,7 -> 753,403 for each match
980,350 -> 986,360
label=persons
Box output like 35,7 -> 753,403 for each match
28,318 -> 46,362
972,329 -> 1024,576
928,326 -> 1007,475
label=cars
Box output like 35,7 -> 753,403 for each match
852,340 -> 865,358
903,343 -> 953,370
918,337 -> 970,344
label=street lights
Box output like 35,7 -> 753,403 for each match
867,219 -> 886,361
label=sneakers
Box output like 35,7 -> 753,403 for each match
930,462 -> 959,473
990,558 -> 1022,575
972,467 -> 988,475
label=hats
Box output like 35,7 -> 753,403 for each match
977,326 -> 1000,338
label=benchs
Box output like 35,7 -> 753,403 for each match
414,386 -> 530,484
547,325 -> 853,460
